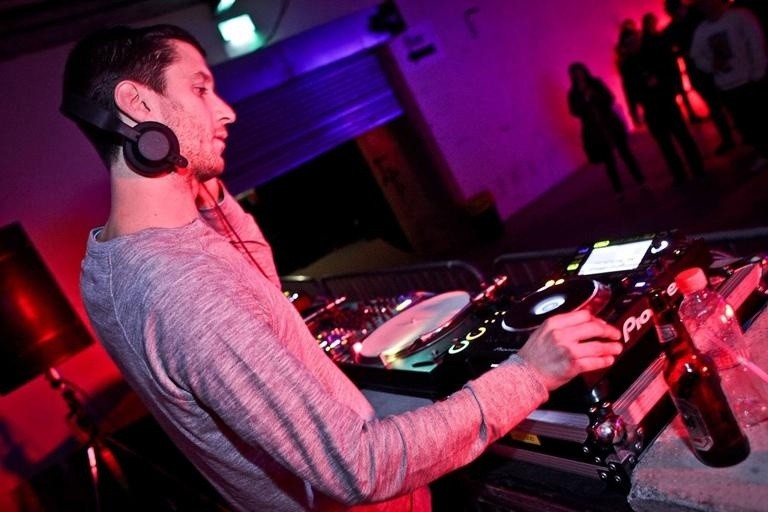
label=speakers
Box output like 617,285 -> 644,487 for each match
0,223 -> 93,396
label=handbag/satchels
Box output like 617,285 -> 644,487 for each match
581,127 -> 614,165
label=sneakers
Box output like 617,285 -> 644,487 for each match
613,181 -> 651,207
671,170 -> 707,192
688,110 -> 705,127
714,138 -> 737,157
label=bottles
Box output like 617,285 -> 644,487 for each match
676,268 -> 766,423
646,285 -> 750,467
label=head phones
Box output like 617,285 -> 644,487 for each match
59,97 -> 180,173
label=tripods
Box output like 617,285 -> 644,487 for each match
44,368 -> 232,512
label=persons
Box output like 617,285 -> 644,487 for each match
57,22 -> 625,510
